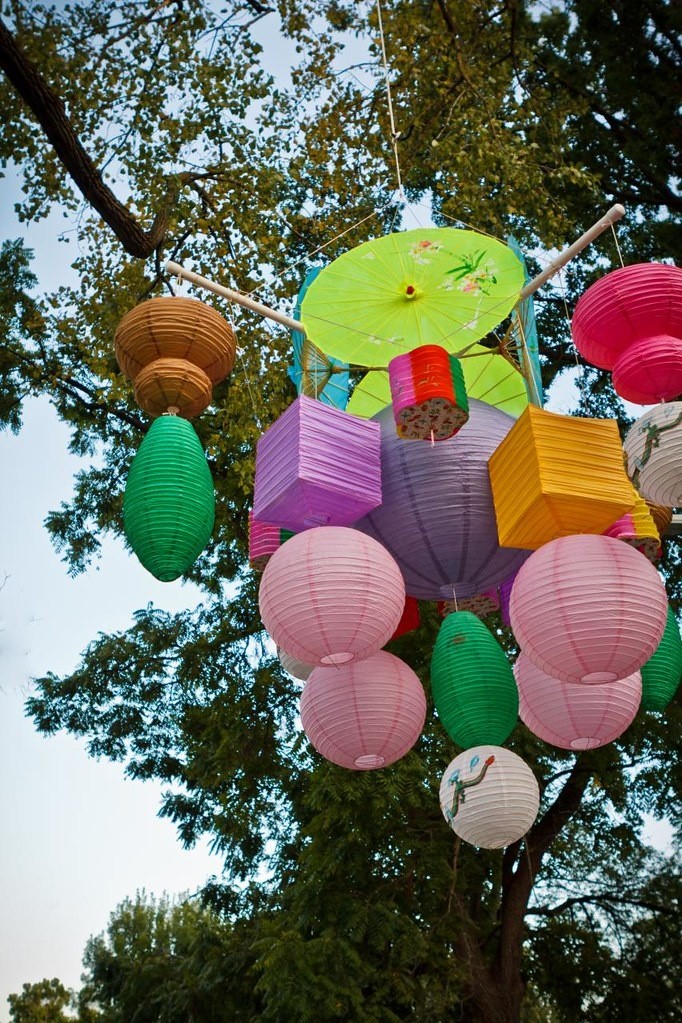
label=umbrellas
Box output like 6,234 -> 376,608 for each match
300,227 -> 524,367
289,267 -> 391,415
458,276 -> 544,412
344,342 -> 528,424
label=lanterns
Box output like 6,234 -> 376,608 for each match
389,593 -> 419,639
500,570 -> 512,626
389,344 -> 469,446
132,359 -> 213,420
612,334 -> 682,406
123,415 -> 216,582
252,395 -> 383,531
114,297 -> 236,389
353,396 -> 537,600
623,401 -> 682,509
571,263 -> 682,371
440,586 -> 498,619
438,745 -> 540,850
246,510 -> 298,569
256,525 -> 406,668
644,498 -> 672,535
486,402 -> 637,550
641,603 -> 682,712
603,484 -> 662,564
513,649 -> 643,751
429,612 -> 519,749
279,645 -> 315,680
300,649 -> 426,771
507,534 -> 669,686
